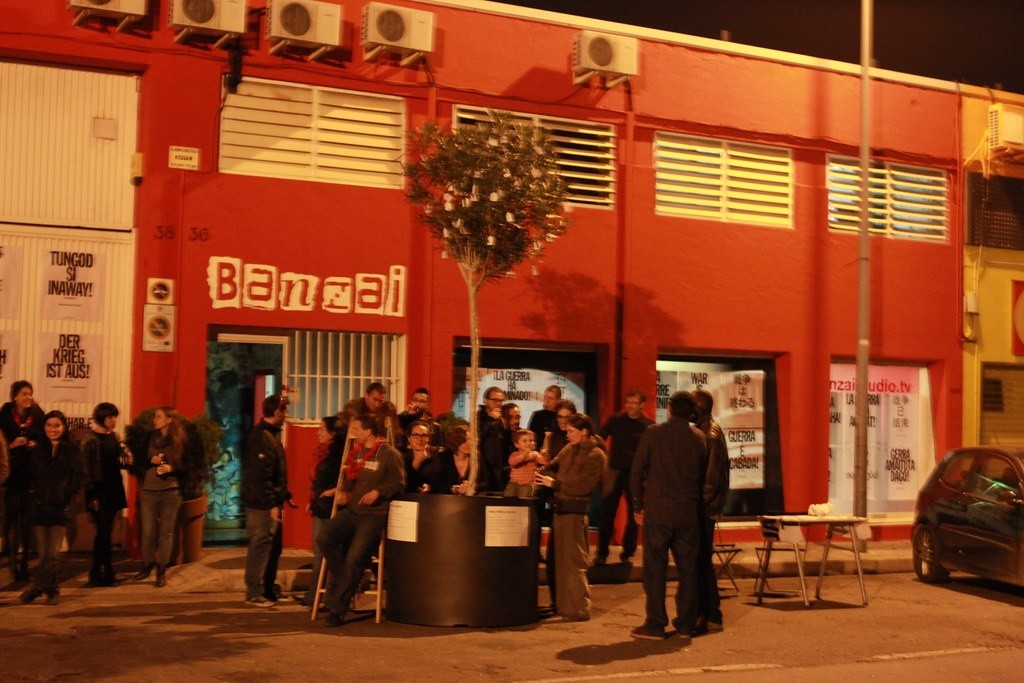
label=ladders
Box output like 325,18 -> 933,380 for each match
311,416 -> 394,624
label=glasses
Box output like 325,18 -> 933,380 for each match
411,433 -> 429,440
557,414 -> 570,420
488,398 -> 505,405
507,415 -> 521,421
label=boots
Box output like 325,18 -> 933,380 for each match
18,556 -> 63,604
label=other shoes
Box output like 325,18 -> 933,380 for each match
561,610 -> 590,622
88,569 -> 119,586
270,592 -> 293,601
244,596 -> 274,607
324,614 -> 343,628
630,627 -> 665,640
696,618 -> 723,633
134,560 -> 155,580
310,605 -> 330,612
155,565 -> 167,586
591,555 -> 607,566
619,551 -> 634,567
673,616 -> 691,639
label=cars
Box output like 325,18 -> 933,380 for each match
910,443 -> 1024,590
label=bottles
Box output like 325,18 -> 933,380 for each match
157,453 -> 166,478
19,424 -> 26,448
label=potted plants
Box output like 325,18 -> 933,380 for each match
375,102 -> 570,629
123,407 -> 222,563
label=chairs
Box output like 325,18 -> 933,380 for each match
711,522 -> 743,595
753,514 -> 809,607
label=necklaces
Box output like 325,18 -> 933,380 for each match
346,436 -> 384,479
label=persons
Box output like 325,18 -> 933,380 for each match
132,406 -> 193,587
240,395 -> 298,608
597,391 -> 657,568
0,381 -> 132,606
630,389 -> 730,641
295,383 -> 605,628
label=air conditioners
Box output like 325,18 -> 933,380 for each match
987,103 -> 1024,154
360,0 -> 438,56
168,0 -> 249,40
572,30 -> 637,76
67,0 -> 147,22
265,0 -> 340,52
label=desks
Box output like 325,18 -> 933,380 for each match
759,515 -> 869,606
